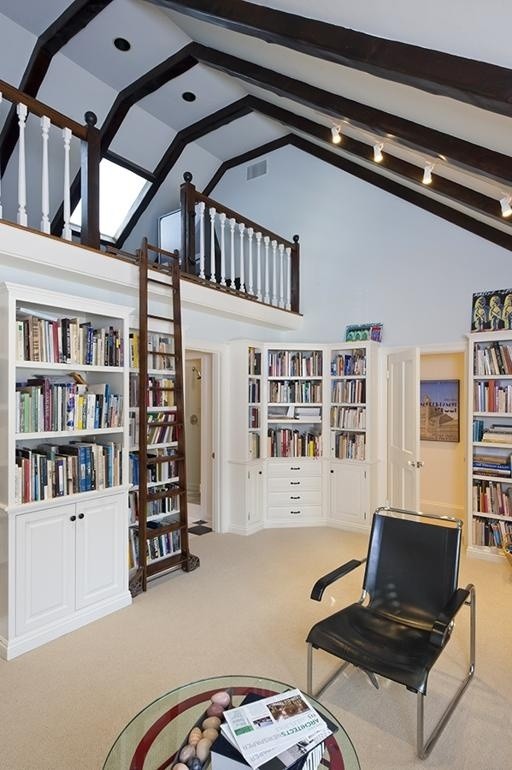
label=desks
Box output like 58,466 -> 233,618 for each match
100,673 -> 362,769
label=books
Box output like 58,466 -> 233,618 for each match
248,347 -> 368,463
472,341 -> 512,553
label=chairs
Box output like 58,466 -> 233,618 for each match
309,506 -> 484,758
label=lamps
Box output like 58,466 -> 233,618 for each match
373,140 -> 386,163
422,160 -> 436,185
330,122 -> 342,143
492,189 -> 512,220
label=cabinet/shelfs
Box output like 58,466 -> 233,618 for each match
0,486 -> 131,663
119,320 -> 184,581
462,329 -> 512,568
2,281 -> 132,507
226,340 -> 385,535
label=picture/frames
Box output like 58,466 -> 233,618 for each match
420,378 -> 461,443
